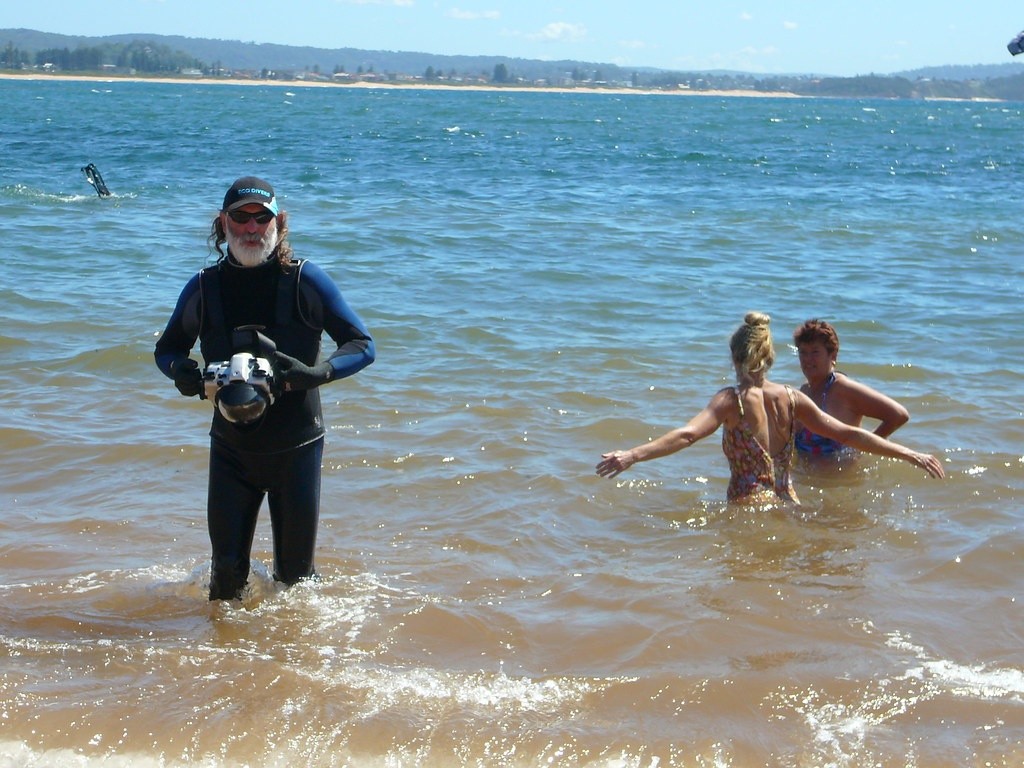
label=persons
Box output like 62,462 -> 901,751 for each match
154,177 -> 375,601
793,318 -> 909,457
596,311 -> 945,501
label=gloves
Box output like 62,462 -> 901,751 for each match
275,350 -> 336,393
172,358 -> 201,396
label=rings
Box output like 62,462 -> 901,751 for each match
937,465 -> 939,467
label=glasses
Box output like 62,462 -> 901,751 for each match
223,210 -> 274,224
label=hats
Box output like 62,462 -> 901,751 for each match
223,177 -> 280,218
